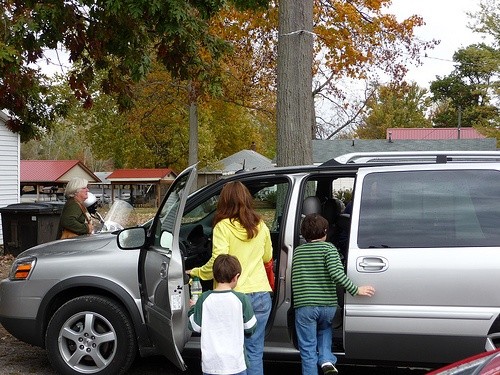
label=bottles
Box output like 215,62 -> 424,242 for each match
191,276 -> 202,302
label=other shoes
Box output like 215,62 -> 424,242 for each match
320,362 -> 339,375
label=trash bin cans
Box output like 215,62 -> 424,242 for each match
0,203 -> 62,255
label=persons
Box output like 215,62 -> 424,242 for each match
184,179 -> 272,375
188,253 -> 257,375
55,177 -> 94,240
291,213 -> 375,375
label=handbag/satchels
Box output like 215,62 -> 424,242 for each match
59,230 -> 78,240
